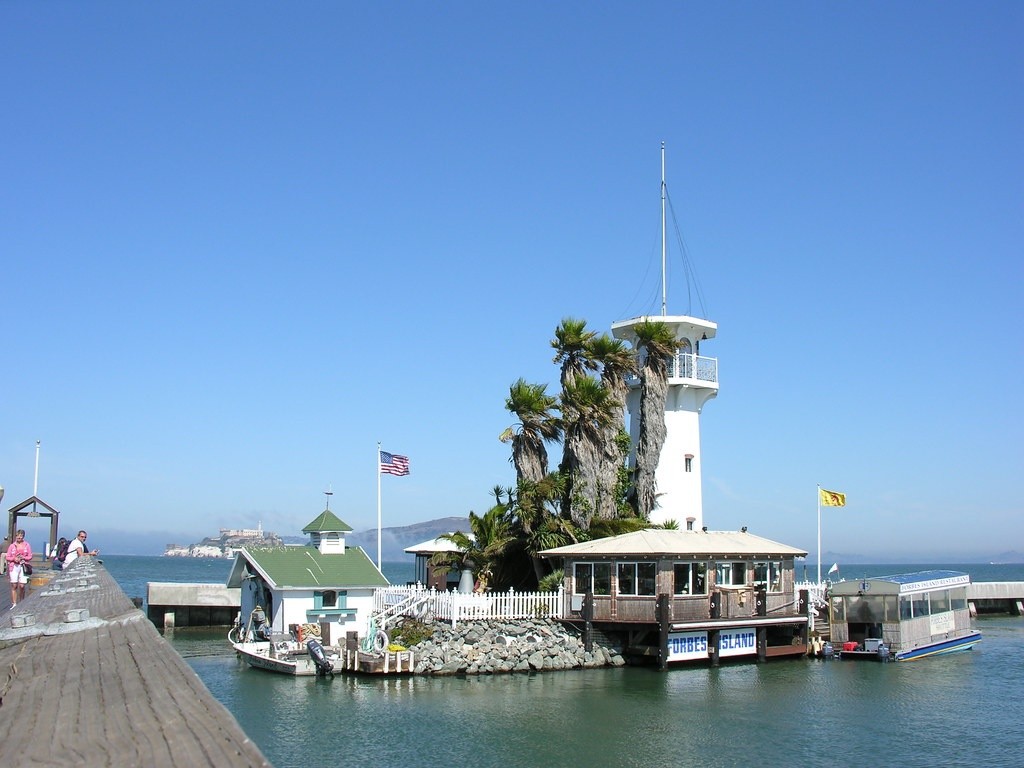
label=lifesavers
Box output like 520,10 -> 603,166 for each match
373,630 -> 389,653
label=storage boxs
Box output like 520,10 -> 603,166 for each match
843,641 -> 857,651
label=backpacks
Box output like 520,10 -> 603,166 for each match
56,538 -> 77,562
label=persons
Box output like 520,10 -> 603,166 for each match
0,537 -> 11,576
5,529 -> 32,610
473,573 -> 488,594
51,531 -> 100,571
857,596 -> 925,621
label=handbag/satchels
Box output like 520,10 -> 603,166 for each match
53,560 -> 64,570
21,563 -> 33,575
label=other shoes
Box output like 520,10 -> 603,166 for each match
9,603 -> 17,610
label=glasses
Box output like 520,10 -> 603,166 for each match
81,536 -> 87,538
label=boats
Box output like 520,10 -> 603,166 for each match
825,568 -> 983,663
226,603 -> 345,678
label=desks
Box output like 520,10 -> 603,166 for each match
864,638 -> 884,652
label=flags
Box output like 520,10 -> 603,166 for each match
821,489 -> 846,507
380,451 -> 410,476
828,563 -> 838,574
